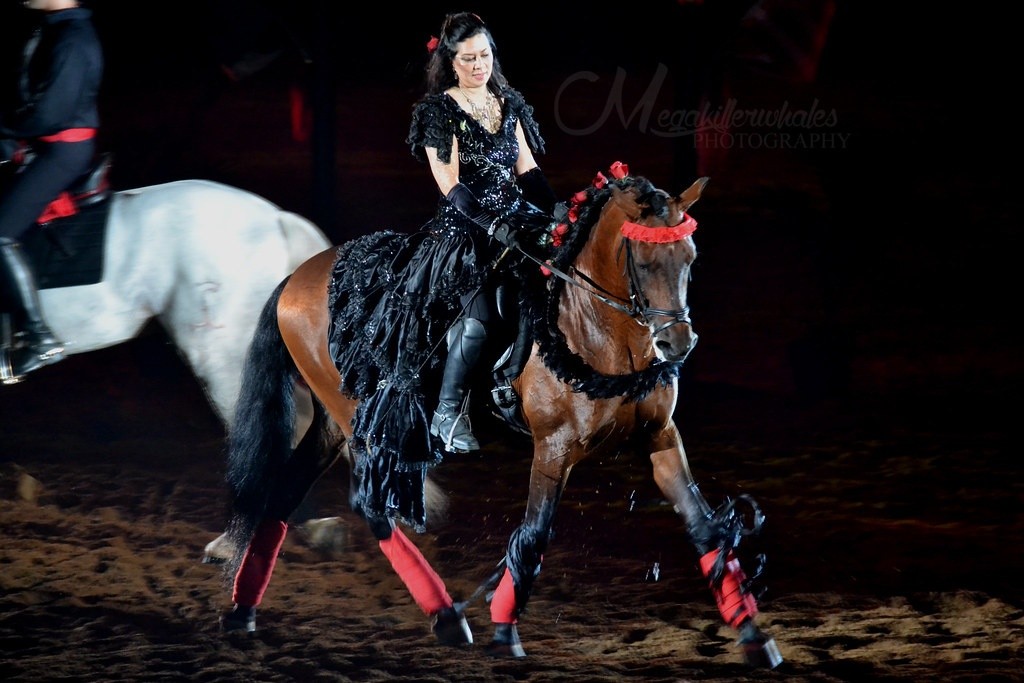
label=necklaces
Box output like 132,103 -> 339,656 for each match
459,85 -> 498,132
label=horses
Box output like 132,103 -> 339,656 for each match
1,179 -> 351,566
218,176 -> 784,670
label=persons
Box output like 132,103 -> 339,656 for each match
407,12 -> 566,452
0,0 -> 105,380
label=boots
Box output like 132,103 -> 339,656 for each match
0,244 -> 65,377
430,316 -> 488,451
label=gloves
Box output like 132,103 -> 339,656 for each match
493,222 -> 524,251
553,201 -> 569,220
